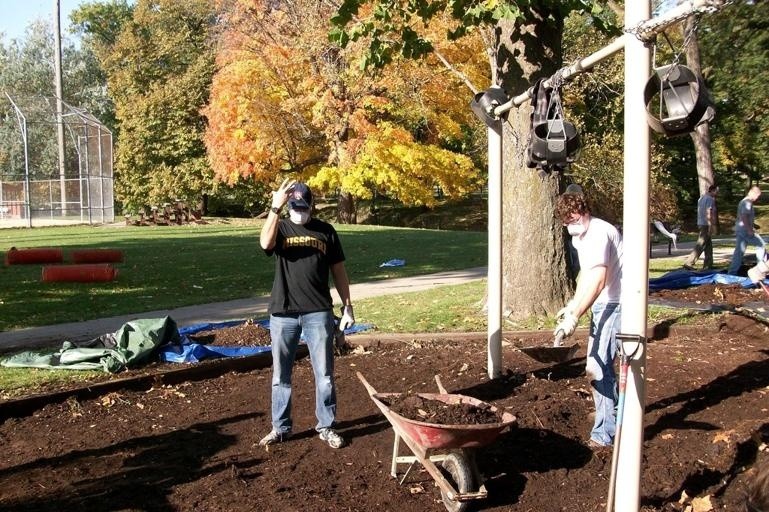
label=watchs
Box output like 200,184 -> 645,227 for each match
270,205 -> 281,214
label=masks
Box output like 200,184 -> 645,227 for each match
289,209 -> 310,225
567,221 -> 587,236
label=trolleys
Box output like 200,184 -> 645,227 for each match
354,370 -> 521,511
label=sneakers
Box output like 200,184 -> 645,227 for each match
319,429 -> 345,449
259,430 -> 288,446
683,264 -> 697,271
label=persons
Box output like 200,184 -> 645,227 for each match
728,186 -> 768,276
259,177 -> 355,448
553,193 -> 623,448
684,185 -> 720,271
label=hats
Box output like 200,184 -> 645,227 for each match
287,183 -> 311,208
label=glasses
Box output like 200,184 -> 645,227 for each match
561,219 -> 577,227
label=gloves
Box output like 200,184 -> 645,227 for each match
339,304 -> 355,331
552,306 -> 578,337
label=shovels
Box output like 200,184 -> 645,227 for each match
521,337 -> 580,363
